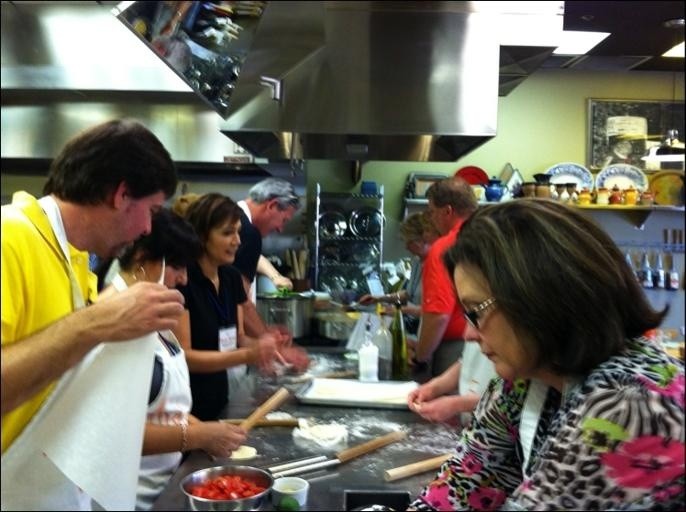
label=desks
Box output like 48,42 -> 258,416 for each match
146,342 -> 468,512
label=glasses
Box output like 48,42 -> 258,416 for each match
459,293 -> 496,331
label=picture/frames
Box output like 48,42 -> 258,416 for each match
583,94 -> 686,177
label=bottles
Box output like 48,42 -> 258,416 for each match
389,301 -> 409,380
358,322 -> 379,383
522,174 -> 557,199
623,247 -> 679,292
374,303 -> 393,382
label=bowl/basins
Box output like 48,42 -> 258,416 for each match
181,464 -> 277,511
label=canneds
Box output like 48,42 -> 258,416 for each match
596,187 -> 609,204
624,189 -> 636,205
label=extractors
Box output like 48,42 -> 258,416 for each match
218,1 -> 501,164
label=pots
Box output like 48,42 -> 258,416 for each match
253,289 -> 316,339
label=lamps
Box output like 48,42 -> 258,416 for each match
640,59 -> 686,168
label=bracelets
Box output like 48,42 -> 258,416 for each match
180,422 -> 187,452
386,291 -> 394,303
396,288 -> 401,305
270,273 -> 281,282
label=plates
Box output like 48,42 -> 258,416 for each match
595,163 -> 649,193
545,161 -> 594,193
649,170 -> 685,206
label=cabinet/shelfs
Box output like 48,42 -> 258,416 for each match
311,179 -> 385,296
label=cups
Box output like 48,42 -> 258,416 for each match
273,476 -> 310,509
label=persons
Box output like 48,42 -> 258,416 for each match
90,208 -> 246,510
177,192 -> 286,420
233,266 -> 310,378
411,178 -> 477,377
345,197 -> 686,511
361,210 -> 440,339
0,121 -> 185,511
406,325 -> 497,429
234,179 -> 301,293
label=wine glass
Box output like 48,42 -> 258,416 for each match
552,181 -> 576,204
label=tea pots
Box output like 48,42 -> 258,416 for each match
478,175 -> 508,202
576,183 -> 655,205
